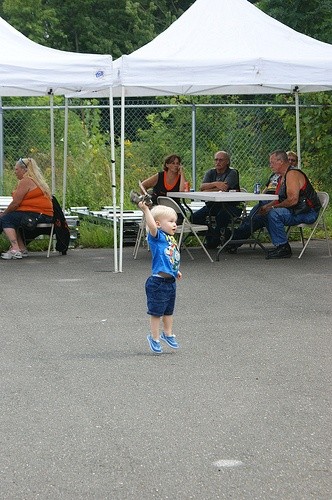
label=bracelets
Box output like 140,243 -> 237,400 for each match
271,205 -> 274,209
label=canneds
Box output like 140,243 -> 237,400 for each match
184,180 -> 190,192
254,183 -> 261,194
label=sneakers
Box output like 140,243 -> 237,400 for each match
269,244 -> 292,258
147,335 -> 162,353
1,249 -> 22,260
21,248 -> 28,258
160,331 -> 179,349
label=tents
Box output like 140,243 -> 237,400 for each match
0,0 -> 332,274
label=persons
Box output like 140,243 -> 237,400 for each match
138,201 -> 182,354
221,151 -> 298,252
0,157 -> 54,260
139,155 -> 190,226
189,151 -> 241,252
259,150 -> 322,259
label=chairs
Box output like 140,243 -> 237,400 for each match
17,196 -> 70,259
132,187 -> 331,261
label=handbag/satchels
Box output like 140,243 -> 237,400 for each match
20,214 -> 38,231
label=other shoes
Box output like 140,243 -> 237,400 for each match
220,227 -> 237,253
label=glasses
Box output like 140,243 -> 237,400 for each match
19,158 -> 27,169
289,159 -> 297,162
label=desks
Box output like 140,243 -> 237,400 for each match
166,192 -> 279,260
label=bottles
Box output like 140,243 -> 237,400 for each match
254,181 -> 261,194
184,177 -> 190,192
